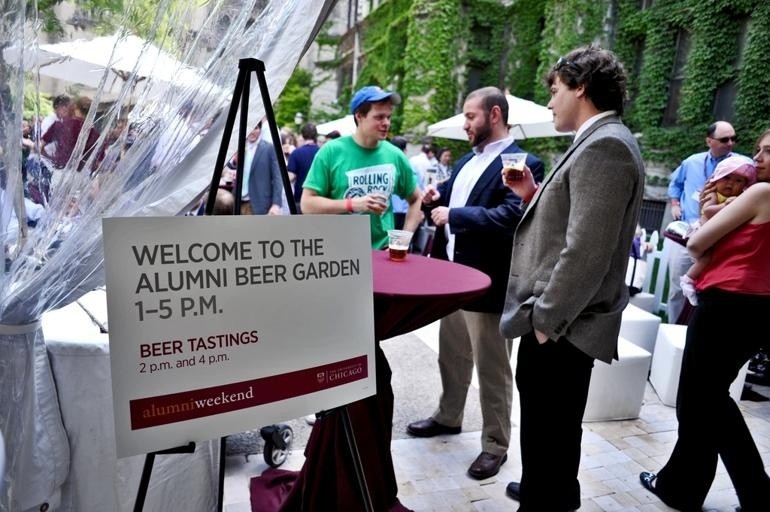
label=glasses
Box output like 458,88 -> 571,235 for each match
711,135 -> 738,144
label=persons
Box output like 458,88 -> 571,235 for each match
203,119 -> 340,214
640,120 -> 769,510
19,93 -> 140,222
389,136 -> 454,230
300,86 -> 426,248
406,86 -> 544,480
500,44 -> 645,511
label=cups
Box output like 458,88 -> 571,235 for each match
500,152 -> 528,182
389,230 -> 414,261
367,190 -> 390,216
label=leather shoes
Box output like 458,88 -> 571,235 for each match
406,418 -> 462,438
468,451 -> 507,481
505,482 -> 521,501
639,471 -> 658,494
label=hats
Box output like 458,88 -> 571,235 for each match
710,156 -> 757,185
350,85 -> 402,115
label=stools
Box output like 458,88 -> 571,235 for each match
580,335 -> 651,424
648,323 -> 750,410
618,302 -> 662,377
629,289 -> 655,313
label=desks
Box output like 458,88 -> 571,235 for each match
27,290 -> 225,512
250,249 -> 493,512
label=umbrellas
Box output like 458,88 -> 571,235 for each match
2,32 -> 231,108
